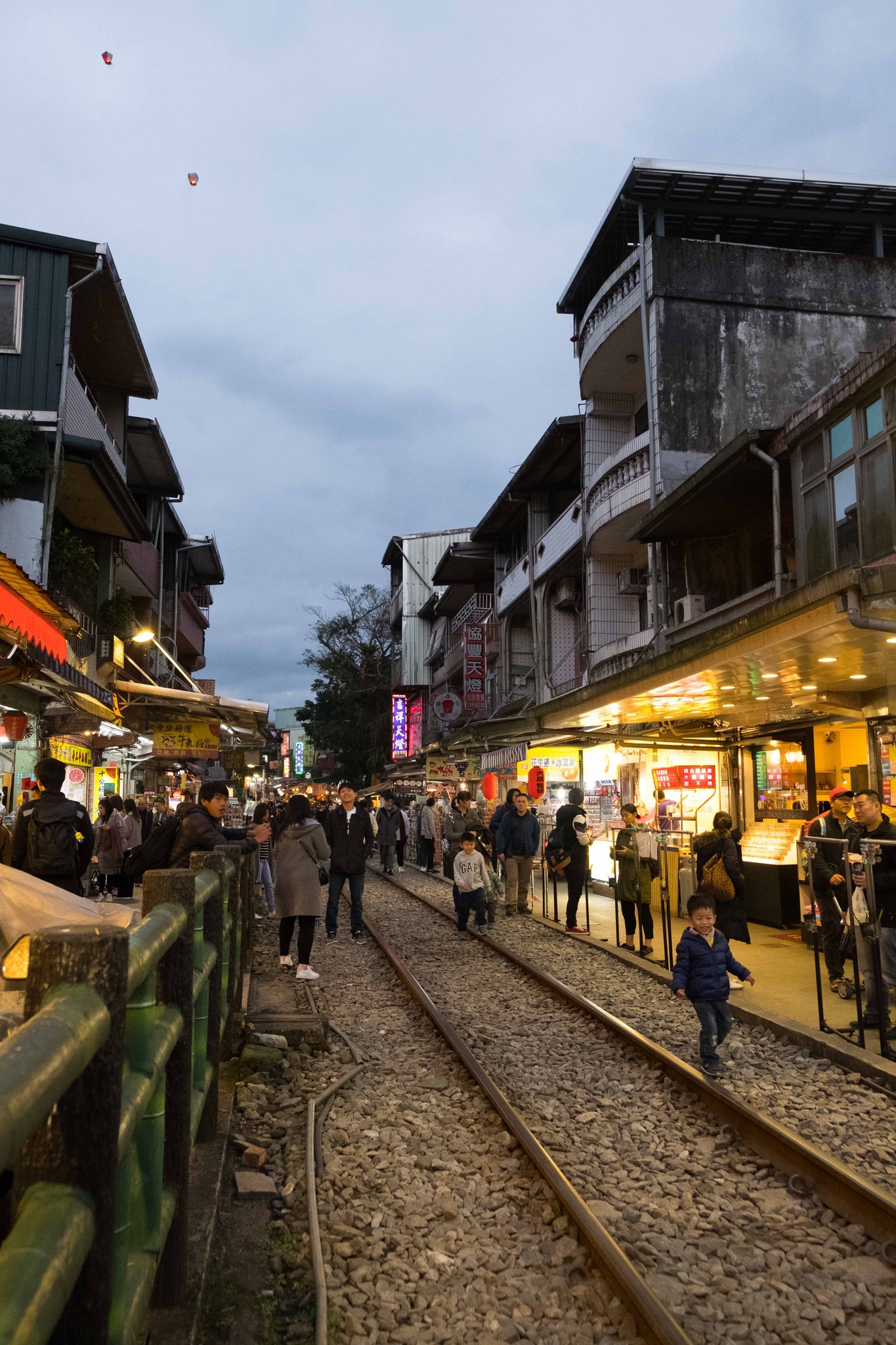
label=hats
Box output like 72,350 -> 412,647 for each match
829,787 -> 854,800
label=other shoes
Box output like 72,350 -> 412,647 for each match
501,875 -> 506,882
637,945 -> 654,955
728,978 -> 744,989
381,865 -> 443,877
827,974 -> 896,1040
451,898 -> 535,937
620,943 -> 635,951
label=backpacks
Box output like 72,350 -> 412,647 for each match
25,801 -> 79,875
545,813 -> 577,875
617,830 -> 663,881
124,812 -> 195,883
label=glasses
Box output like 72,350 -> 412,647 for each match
836,798 -> 854,803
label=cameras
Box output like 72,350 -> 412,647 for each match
153,821 -> 162,827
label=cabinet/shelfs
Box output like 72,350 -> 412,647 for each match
760,771 -> 838,810
737,821 -> 852,953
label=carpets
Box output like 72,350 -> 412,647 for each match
767,932 -> 806,944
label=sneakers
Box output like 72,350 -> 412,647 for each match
701,1058 -> 723,1077
324,926 -> 367,945
253,909 -> 277,919
296,965 -> 319,979
106,893 -> 113,902
279,954 -> 293,966
566,924 -> 590,935
94,892 -> 104,902
114,896 -> 132,899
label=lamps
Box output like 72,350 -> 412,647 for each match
122,630 -> 155,643
785,743 -> 804,763
841,777 -> 847,784
92,730 -> 112,740
445,755 -> 477,765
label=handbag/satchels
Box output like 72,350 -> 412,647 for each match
700,837 -> 746,900
846,887 -> 874,927
319,866 -> 329,886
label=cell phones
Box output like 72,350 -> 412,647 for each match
97,825 -> 107,829
591,836 -> 598,839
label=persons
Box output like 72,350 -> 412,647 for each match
671,894 -> 754,1077
0,758 -> 896,1028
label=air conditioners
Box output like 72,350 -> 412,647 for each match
674,595 -> 705,626
555,577 -> 574,607
616,567 -> 647,595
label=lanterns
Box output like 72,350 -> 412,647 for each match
482,772 -> 498,801
528,766 -> 546,803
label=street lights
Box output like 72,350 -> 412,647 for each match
246,763 -> 266,803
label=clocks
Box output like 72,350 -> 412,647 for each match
69,768 -> 84,784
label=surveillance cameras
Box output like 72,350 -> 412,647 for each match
823,732 -> 830,737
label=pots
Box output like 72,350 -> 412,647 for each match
821,785 -> 829,790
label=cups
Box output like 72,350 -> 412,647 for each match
586,869 -> 591,879
666,805 -> 672,816
609,878 -> 615,887
848,855 -> 864,877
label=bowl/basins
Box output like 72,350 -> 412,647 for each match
669,812 -> 674,816
